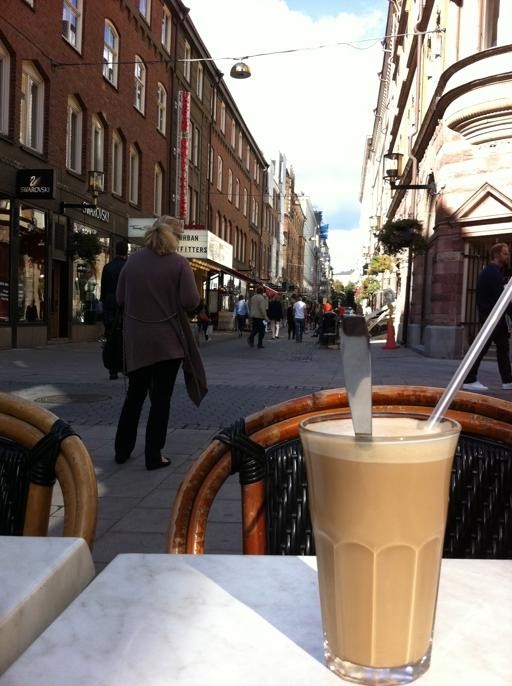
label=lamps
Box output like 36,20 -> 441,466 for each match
383,148 -> 436,194
61,171 -> 106,215
229,59 -> 253,78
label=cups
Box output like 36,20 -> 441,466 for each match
300,409 -> 464,685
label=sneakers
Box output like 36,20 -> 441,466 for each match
462,381 -> 487,392
502,383 -> 512,389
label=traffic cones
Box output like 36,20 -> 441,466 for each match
383,326 -> 400,349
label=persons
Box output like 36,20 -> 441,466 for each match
233,286 -> 355,348
26,299 -> 38,321
462,244 -> 512,391
195,298 -> 211,340
116,214 -> 208,470
101,240 -> 127,379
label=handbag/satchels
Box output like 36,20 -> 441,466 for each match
197,309 -> 209,323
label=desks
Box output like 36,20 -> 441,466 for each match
1,533 -> 95,671
1,552 -> 512,686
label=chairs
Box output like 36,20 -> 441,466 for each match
1,388 -> 97,554
165,383 -> 512,555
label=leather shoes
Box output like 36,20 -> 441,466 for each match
145,456 -> 172,470
